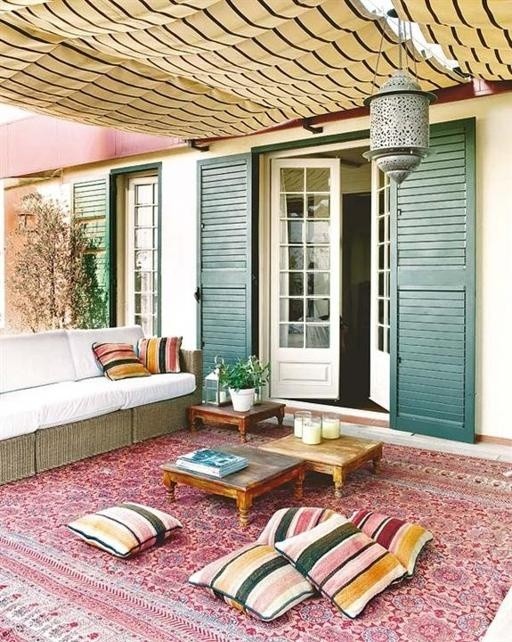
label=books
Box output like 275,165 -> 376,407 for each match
175,446 -> 249,479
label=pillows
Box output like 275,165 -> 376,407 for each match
92,337 -> 183,380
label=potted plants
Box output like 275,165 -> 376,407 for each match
219,357 -> 256,411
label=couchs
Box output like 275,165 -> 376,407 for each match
0,324 -> 202,484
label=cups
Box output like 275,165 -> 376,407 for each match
294,410 -> 341,445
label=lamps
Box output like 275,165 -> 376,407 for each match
360,0 -> 438,185
